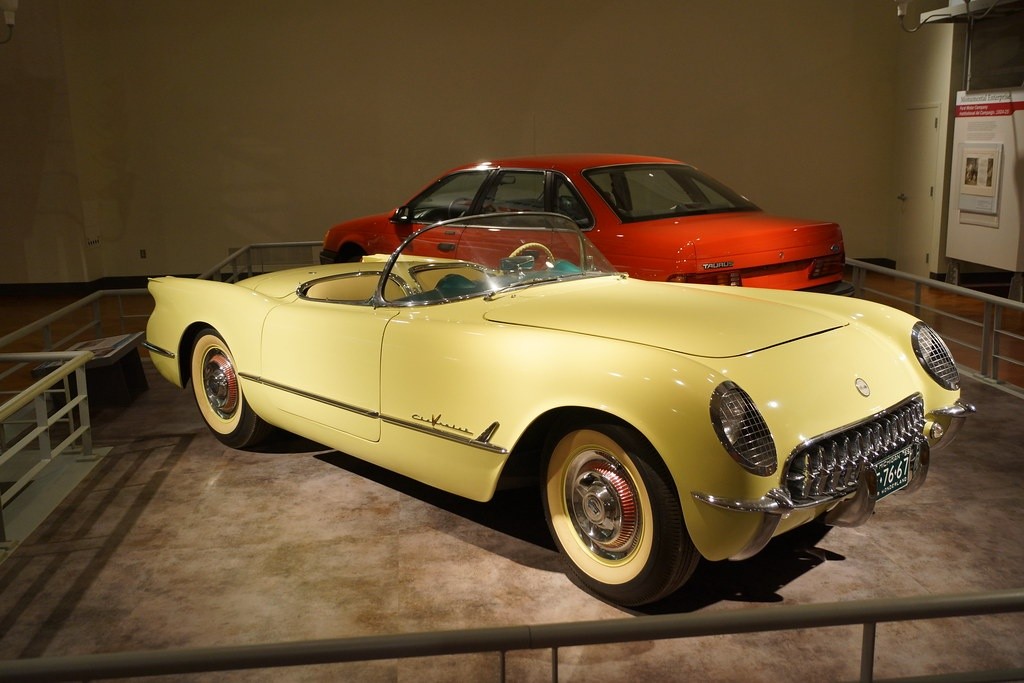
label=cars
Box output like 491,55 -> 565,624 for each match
319,153 -> 856,306
142,212 -> 980,611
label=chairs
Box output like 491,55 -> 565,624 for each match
435,274 -> 476,290
